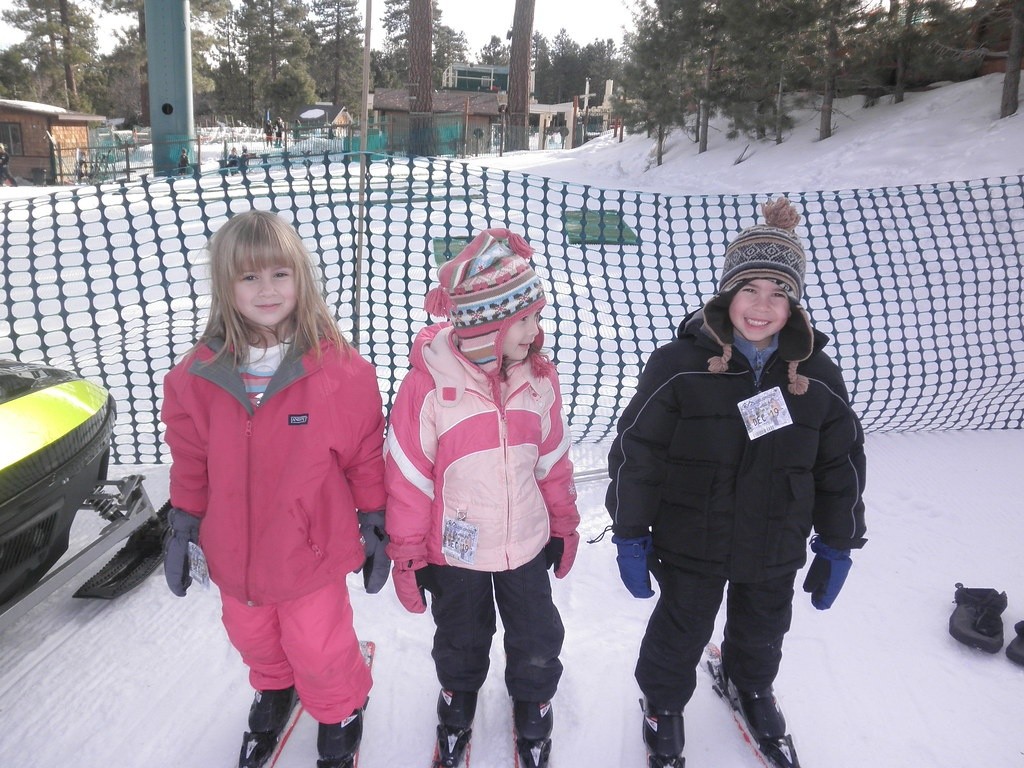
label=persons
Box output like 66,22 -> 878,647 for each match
176,148 -> 189,176
226,145 -> 249,168
160,210 -> 392,760
264,117 -> 301,148
605,199 -> 866,757
322,122 -> 342,142
0,143 -> 18,187
132,127 -> 139,152
75,150 -> 88,177
384,228 -> 581,741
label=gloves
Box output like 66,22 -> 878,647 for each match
612,532 -> 655,598
392,565 -> 428,614
352,509 -> 391,594
162,508 -> 201,598
546,532 -> 581,580
803,535 -> 852,610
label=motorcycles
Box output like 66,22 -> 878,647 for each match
0,356 -> 172,699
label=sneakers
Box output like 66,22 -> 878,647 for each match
317,708 -> 363,758
642,697 -> 686,757
248,684 -> 296,735
513,700 -> 553,740
736,685 -> 786,740
437,688 -> 478,729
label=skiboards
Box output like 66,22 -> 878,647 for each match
431,684 -> 555,768
260,641 -> 377,768
637,638 -> 802,767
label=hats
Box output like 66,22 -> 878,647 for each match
702,199 -> 810,396
423,229 -> 558,406
